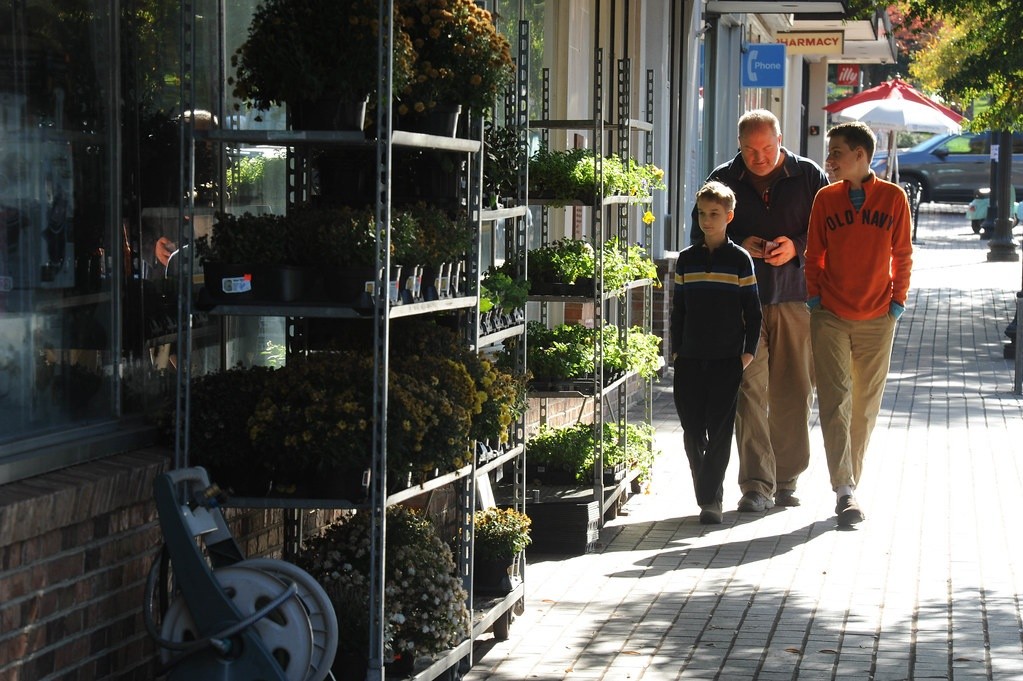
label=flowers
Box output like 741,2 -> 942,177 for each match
181,196 -> 477,272
159,321 -> 532,483
444,505 -> 533,562
228,0 -> 517,122
284,503 -> 475,664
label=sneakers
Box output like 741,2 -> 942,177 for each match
774,488 -> 799,506
835,494 -> 862,526
700,500 -> 723,520
738,491 -> 774,511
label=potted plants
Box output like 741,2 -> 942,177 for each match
494,149 -> 670,502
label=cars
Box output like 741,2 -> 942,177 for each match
870,127 -> 1023,210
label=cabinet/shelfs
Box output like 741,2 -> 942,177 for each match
488,21 -> 654,545
171,0 -> 529,681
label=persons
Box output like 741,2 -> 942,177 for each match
125,109 -> 219,280
690,108 -> 831,512
805,121 -> 913,526
670,181 -> 763,524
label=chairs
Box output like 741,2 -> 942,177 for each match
904,180 -> 923,241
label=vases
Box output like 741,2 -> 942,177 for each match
198,257 -> 462,315
284,84 -> 371,134
396,99 -> 462,137
203,452 -> 465,500
473,552 -> 510,596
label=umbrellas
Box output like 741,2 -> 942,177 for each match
820,72 -> 965,183
